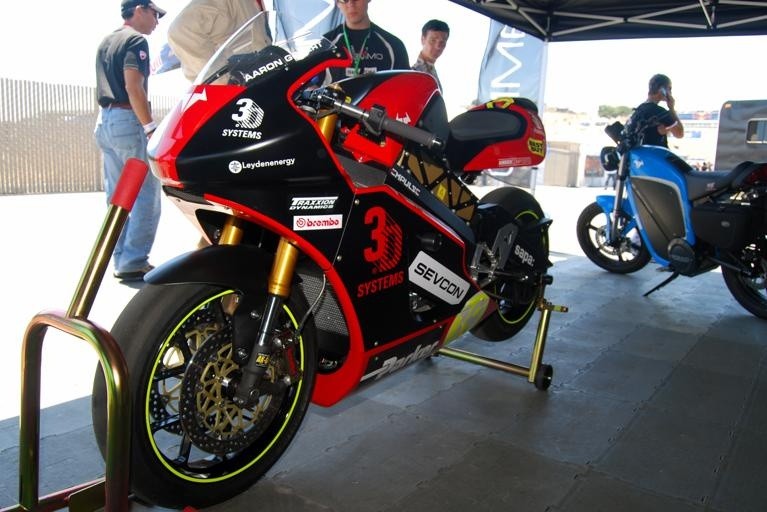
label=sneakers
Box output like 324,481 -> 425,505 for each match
114,266 -> 152,282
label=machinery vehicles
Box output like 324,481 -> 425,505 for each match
714,99 -> 766,171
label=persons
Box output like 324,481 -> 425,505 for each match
93,1 -> 163,280
630,73 -> 684,148
604,172 -> 616,192
309,1 -> 409,73
168,1 -> 272,248
411,20 -> 450,92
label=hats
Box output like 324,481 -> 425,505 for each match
114,0 -> 167,13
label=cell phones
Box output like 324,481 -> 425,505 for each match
660,87 -> 666,98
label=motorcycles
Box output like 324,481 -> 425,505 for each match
576,109 -> 767,320
91,11 -> 554,509
